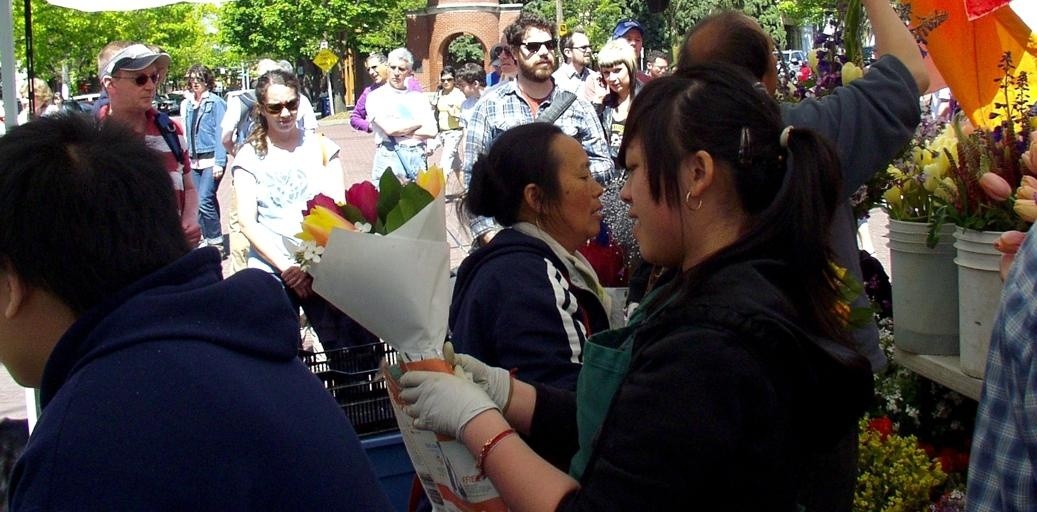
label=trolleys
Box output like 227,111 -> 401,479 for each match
275,272 -> 424,510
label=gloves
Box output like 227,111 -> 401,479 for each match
397,341 -> 510,444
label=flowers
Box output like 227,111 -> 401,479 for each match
772,50 -> 1035,512
280,160 -> 446,276
596,165 -> 655,332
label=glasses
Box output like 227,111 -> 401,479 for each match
574,46 -> 592,50
521,39 -> 557,53
262,95 -> 300,114
112,74 -> 159,87
441,79 -> 454,82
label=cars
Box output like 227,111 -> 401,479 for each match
0,85 -> 195,122
771,45 -> 874,87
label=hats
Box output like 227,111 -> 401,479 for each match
612,18 -> 644,40
98,43 -> 172,80
